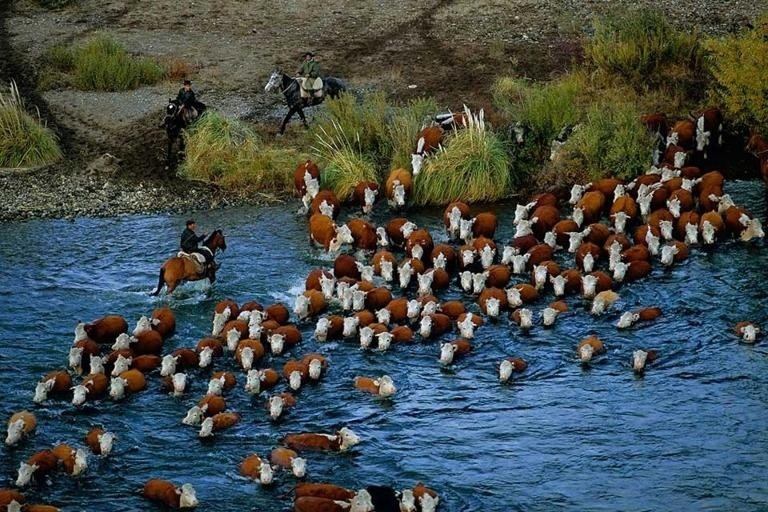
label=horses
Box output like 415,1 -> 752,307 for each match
264,67 -> 346,138
149,229 -> 227,298
162,96 -> 206,170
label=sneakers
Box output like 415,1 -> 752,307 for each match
308,97 -> 314,104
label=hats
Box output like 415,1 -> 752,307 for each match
302,52 -> 315,57
181,80 -> 193,84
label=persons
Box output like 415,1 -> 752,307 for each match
176,80 -> 196,122
295,52 -> 320,105
180,220 -> 216,271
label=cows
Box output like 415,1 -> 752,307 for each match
436,340 -> 471,363
282,426 -> 360,454
32,306 -> 176,407
0,409 -> 119,512
353,375 -> 397,401
277,482 -> 441,512
498,357 -> 526,379
160,299 -> 304,438
143,480 -> 198,510
236,453 -> 280,485
294,109 -> 766,355
270,446 -> 309,479
630,350 -> 658,371
575,336 -> 602,361
